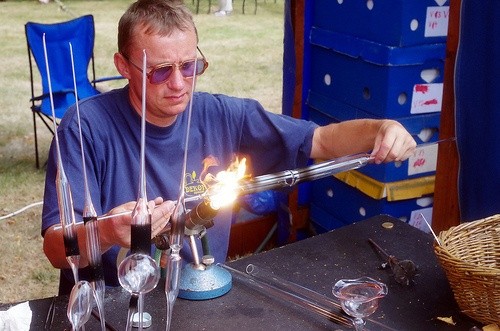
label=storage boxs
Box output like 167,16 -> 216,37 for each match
275,0 -> 500,248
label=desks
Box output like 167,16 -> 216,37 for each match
0,213 -> 488,331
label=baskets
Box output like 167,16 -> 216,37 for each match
435,213 -> 500,325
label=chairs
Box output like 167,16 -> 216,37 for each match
25,14 -> 128,171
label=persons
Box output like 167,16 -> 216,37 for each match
42,0 -> 416,296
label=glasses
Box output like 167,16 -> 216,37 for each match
126,45 -> 209,85
